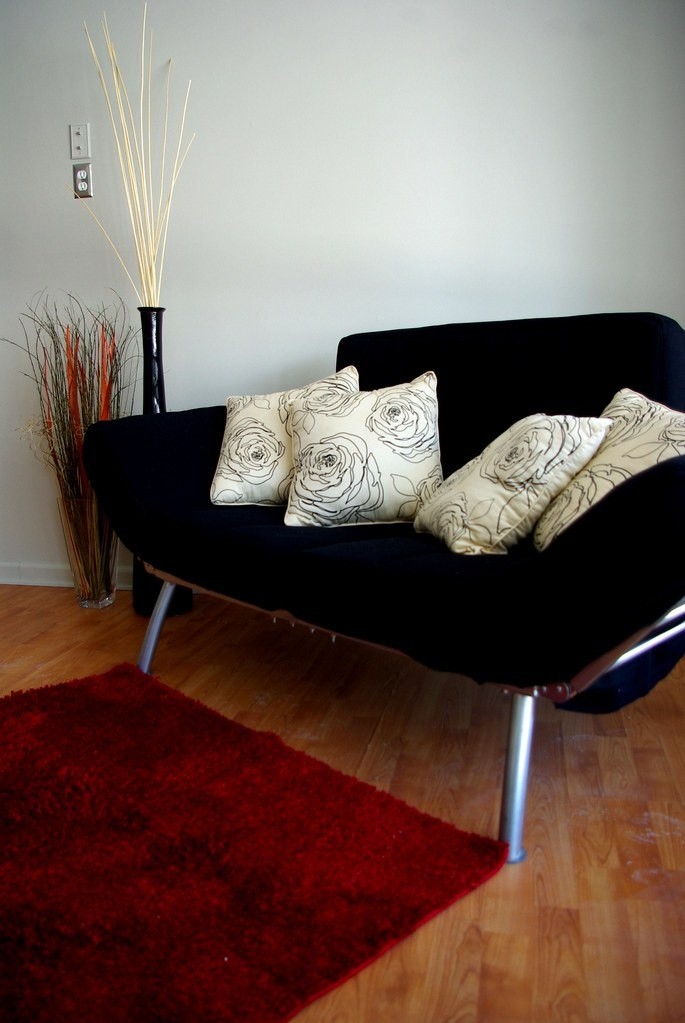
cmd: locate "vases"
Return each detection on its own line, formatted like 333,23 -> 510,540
57,498 -> 119,609
133,307 -> 193,616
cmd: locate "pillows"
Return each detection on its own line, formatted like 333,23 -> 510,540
533,388 -> 685,552
284,371 -> 443,528
209,365 -> 359,507
414,414 -> 613,556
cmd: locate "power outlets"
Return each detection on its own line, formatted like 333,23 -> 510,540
72,163 -> 94,200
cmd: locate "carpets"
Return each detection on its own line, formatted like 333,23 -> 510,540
0,664 -> 509,1022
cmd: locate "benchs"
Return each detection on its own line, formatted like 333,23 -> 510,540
82,312 -> 685,863
